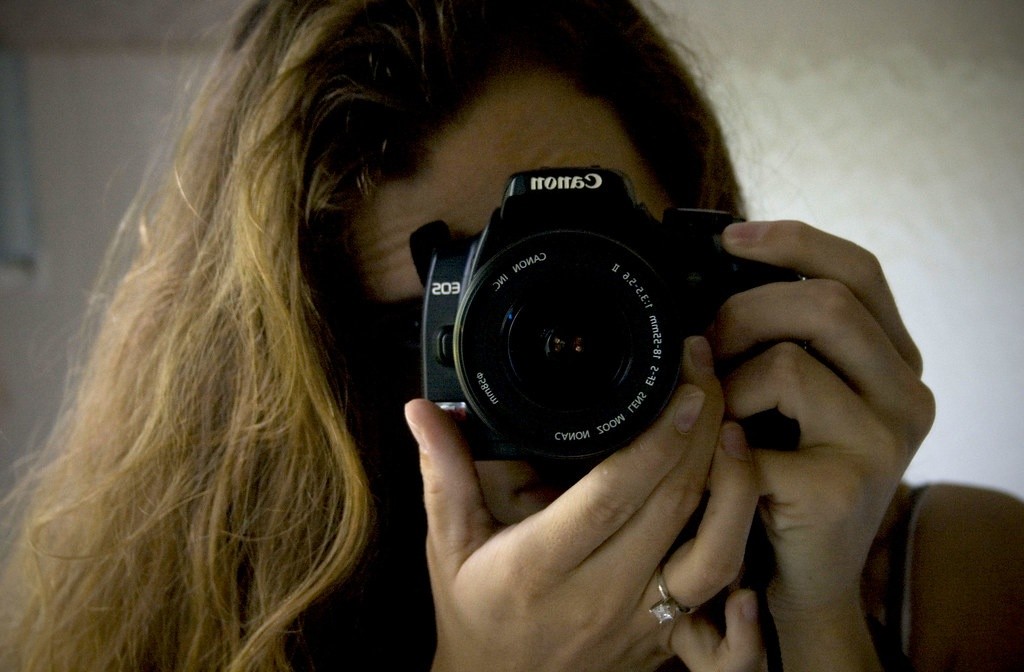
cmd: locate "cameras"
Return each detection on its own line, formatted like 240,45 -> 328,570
418,167 -> 801,466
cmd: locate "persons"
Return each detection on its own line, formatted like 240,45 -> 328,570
0,1 -> 1023,672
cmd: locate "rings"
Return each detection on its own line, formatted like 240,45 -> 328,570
648,565 -> 700,624
